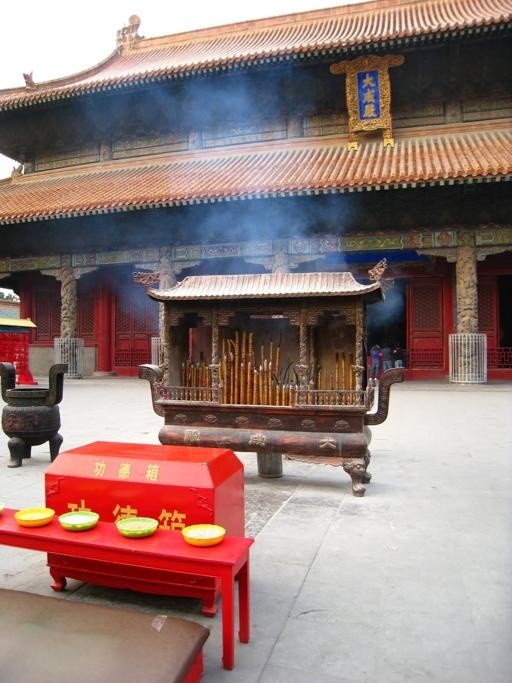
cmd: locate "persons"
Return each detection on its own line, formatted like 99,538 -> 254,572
392,344 -> 403,368
369,344 -> 382,379
382,341 -> 394,372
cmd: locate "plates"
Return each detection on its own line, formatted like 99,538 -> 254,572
13,505 -> 228,548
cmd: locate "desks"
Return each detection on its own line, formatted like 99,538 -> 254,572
1,509 -> 251,668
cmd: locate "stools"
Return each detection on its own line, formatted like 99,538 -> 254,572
1,586 -> 209,678
